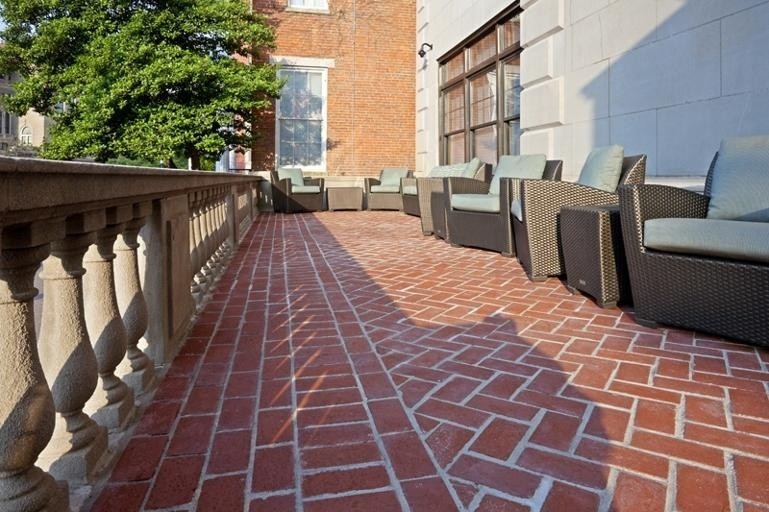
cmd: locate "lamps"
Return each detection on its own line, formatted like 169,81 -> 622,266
417,43 -> 433,58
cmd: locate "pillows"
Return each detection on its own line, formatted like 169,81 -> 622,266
487,154 -> 547,196
705,136 -> 769,222
459,157 -> 482,179
576,144 -> 625,193
380,167 -> 409,187
277,168 -> 304,186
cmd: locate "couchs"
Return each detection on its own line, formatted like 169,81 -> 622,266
270,170 -> 325,215
399,162 -> 487,219
618,150 -> 769,346
509,153 -> 648,282
442,160 -> 564,257
364,170 -> 413,211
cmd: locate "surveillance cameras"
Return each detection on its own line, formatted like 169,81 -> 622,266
419,50 -> 426,57
